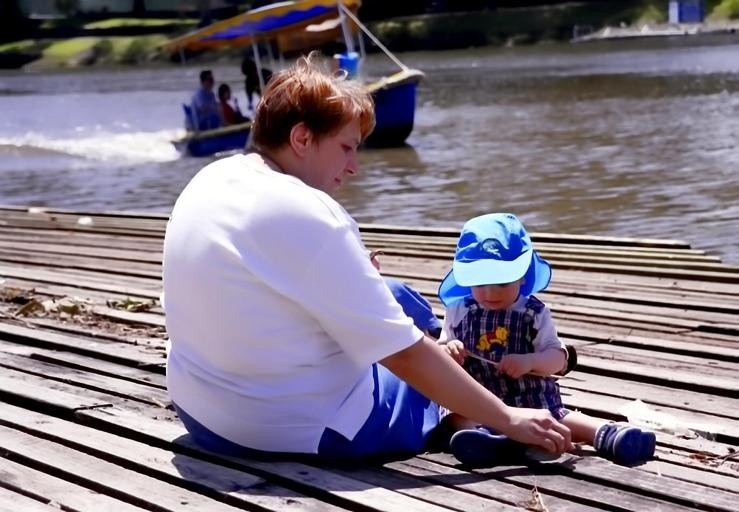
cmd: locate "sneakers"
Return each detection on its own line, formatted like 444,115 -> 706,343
592,419 -> 657,468
449,423 -> 528,469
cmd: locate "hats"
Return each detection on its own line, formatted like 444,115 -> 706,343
452,213 -> 534,288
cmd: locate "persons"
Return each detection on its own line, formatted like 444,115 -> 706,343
435,211 -> 656,468
241,57 -> 273,110
184,70 -> 217,131
218,83 -> 240,125
160,52 -> 579,474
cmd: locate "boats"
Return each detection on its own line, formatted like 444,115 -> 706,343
156,1 -> 425,157
